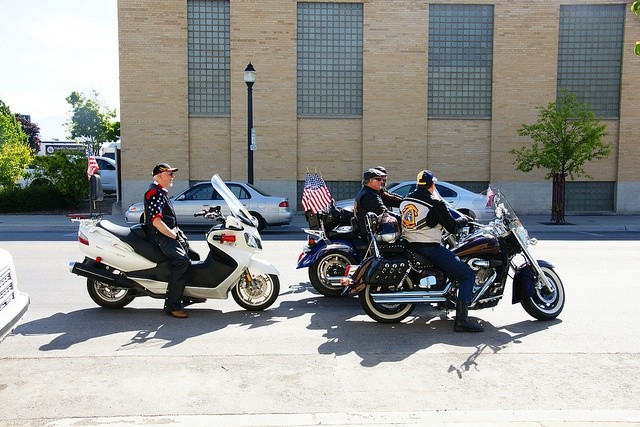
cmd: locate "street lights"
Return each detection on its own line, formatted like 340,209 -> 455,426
242,62 -> 256,185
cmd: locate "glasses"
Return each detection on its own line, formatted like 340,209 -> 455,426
375,178 -> 382,181
168,173 -> 173,176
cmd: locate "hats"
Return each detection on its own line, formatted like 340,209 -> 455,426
416,169 -> 437,185
375,166 -> 387,173
152,163 -> 178,177
364,168 -> 387,179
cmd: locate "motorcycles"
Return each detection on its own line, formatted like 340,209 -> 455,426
296,203 -> 473,295
68,173 -> 280,311
341,187 -> 566,320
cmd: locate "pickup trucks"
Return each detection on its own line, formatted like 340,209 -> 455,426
15,156 -> 117,192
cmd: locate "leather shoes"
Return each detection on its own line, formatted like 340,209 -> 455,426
173,310 -> 188,318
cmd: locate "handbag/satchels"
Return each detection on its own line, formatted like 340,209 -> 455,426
304,198 -> 341,231
363,258 -> 411,288
374,208 -> 400,243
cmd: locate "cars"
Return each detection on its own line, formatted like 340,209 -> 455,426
335,179 -> 495,222
124,180 -> 293,234
0,246 -> 32,344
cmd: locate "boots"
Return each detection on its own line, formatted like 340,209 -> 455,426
453,299 -> 485,332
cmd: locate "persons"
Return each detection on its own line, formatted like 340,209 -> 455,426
398,169 -> 485,333
352,168 -> 400,246
376,165 -> 404,211
142,162 -> 208,319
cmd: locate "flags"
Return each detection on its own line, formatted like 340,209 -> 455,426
308,173 -> 332,215
302,174 -> 313,213
86,144 -> 100,180
320,176 -> 330,214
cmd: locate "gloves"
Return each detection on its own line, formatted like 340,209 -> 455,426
176,233 -> 187,251
178,230 -> 189,248
